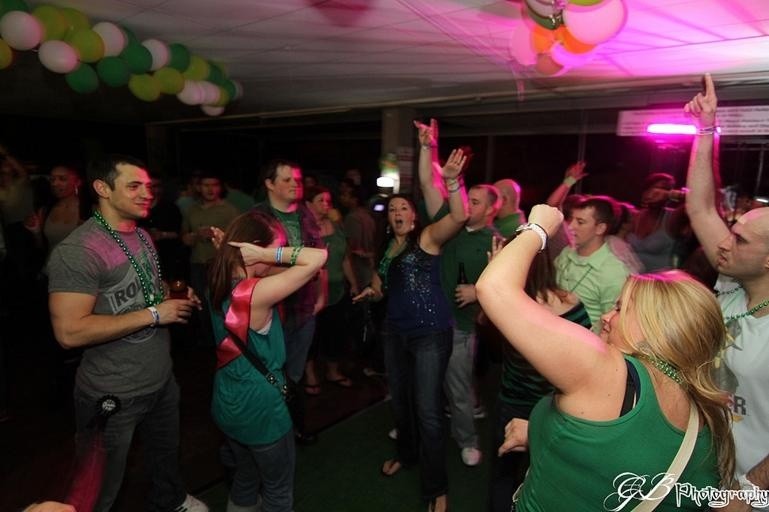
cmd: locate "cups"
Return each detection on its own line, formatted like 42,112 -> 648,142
168,281 -> 189,299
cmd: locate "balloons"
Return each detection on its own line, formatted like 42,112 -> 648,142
0,0 -> 244,117
506,1 -> 624,77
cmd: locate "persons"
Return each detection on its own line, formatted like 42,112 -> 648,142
683,71 -> 768,512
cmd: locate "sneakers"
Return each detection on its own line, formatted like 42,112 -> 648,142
443,403 -> 488,420
175,495 -> 209,512
461,447 -> 480,466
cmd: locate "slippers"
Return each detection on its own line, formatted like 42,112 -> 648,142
305,384 -> 320,397
333,377 -> 354,388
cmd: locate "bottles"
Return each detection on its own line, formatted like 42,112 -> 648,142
456,264 -> 467,304
